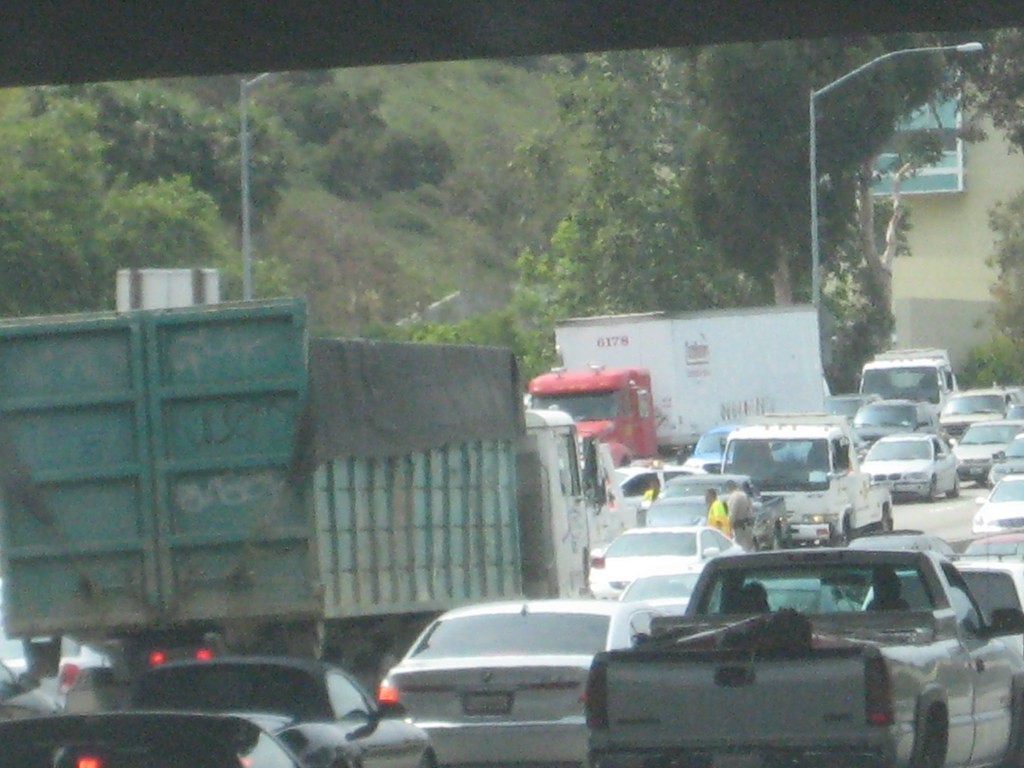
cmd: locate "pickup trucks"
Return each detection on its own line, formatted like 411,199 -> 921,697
580,542 -> 1024,768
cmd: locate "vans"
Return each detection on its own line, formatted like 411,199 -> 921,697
823,394 -> 884,426
851,398 -> 944,463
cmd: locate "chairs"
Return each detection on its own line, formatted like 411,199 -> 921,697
807,443 -> 837,472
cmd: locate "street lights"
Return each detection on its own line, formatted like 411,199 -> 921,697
810,41 -> 987,306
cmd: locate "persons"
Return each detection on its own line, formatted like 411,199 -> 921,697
726,479 -> 757,555
704,489 -> 731,540
639,484 -> 655,509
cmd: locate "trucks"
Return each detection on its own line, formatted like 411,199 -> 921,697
642,474 -> 790,551
720,411 -> 895,551
1,292 -> 606,659
856,347 -> 962,417
524,300 -> 830,478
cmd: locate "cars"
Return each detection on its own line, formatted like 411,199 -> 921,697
854,558 -> 1024,642
117,654 -> 440,768
970,471 -> 1024,535
859,444 -> 963,502
604,568 -> 830,656
374,598 -> 664,768
937,385 -> 1024,484
819,535 -> 960,611
613,466 -> 709,526
689,425 -> 743,472
0,631 -> 114,723
585,525 -> 749,597
1,709 -> 309,767
953,534 -> 1024,564
